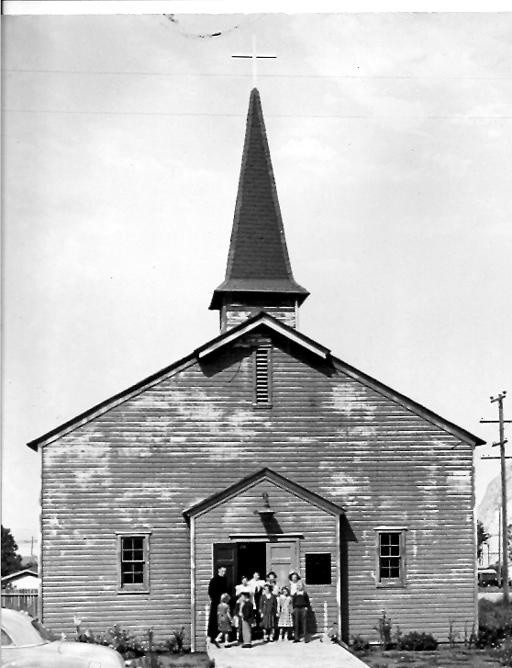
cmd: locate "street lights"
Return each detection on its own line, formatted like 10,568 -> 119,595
484,542 -> 489,566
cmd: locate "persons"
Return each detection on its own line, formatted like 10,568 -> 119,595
206,564 -> 311,648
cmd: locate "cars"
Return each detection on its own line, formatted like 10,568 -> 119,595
0,607 -> 126,668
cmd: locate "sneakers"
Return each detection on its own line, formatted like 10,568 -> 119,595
209,635 -> 310,648
